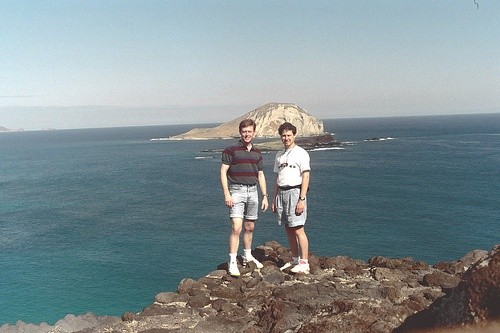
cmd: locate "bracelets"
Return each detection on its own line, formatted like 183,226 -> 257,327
262,194 -> 268,196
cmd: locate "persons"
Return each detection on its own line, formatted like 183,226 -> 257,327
272,122 -> 312,274
220,119 -> 269,276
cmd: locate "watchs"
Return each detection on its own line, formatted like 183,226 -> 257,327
299,196 -> 305,200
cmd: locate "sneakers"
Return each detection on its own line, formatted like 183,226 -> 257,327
279,256 -> 300,271
291,259 -> 310,274
242,256 -> 263,268
227,261 -> 241,277
